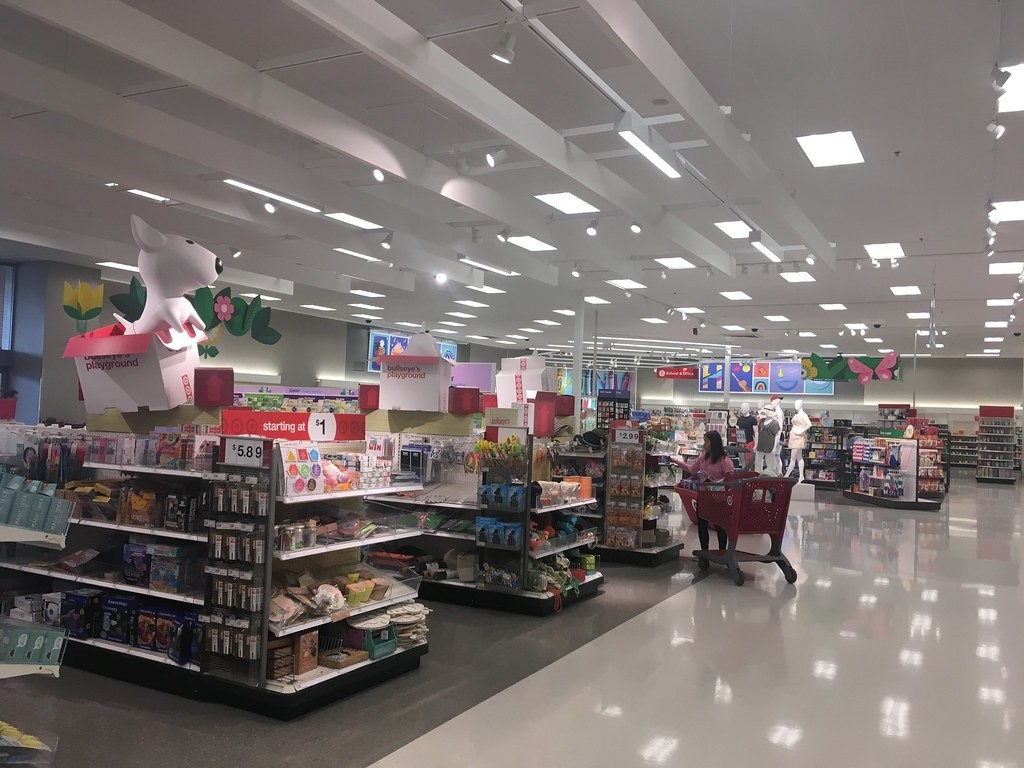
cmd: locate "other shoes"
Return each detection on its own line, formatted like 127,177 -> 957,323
690,556 -> 699,561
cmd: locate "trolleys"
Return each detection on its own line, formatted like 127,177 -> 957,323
674,470 -> 798,585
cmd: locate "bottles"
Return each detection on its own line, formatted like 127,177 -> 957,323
281,523 -> 316,551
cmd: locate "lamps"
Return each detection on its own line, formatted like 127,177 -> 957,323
658,271 -> 668,281
706,267 -> 715,278
586,213 -> 602,237
623,291 -> 631,299
485,147 -> 508,167
982,66 -> 1024,325
570,261 -> 585,278
263,202 -> 279,214
380,231 -> 394,250
794,264 -> 802,274
497,226 -> 511,243
761,263 -> 771,276
805,253 -> 901,272
776,263 -> 782,273
741,266 -> 750,276
630,221 -> 644,234
470,226 -> 485,244
228,246 -> 242,258
664,307 -> 708,330
785,329 -> 949,336
540,342 -> 757,372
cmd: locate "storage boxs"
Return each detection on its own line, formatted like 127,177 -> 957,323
495,355 -> 559,409
121,541 -> 205,597
14,592 -> 46,612
130,603 -> 204,668
314,554 -> 391,609
449,387 -> 480,415
534,392 -> 575,436
512,402 -> 535,434
99,595 -> 133,646
62,587 -> 104,640
62,323 -> 208,415
478,392 -> 497,416
358,383 -> 379,410
194,367 -> 235,408
164,493 -> 197,533
367,355 -> 455,413
268,647 -> 293,677
41,594 -> 62,628
10,608 -> 43,622
342,545 -> 423,599
293,630 -> 319,675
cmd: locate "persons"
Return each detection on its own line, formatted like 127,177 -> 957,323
758,394 -> 785,476
783,399 -> 812,483
668,430 -> 736,564
730,403 -> 758,469
754,404 -> 781,476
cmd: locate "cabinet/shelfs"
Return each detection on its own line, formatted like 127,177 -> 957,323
899,424 -> 950,499
1014,427 -> 1022,467
950,434 -> 977,465
802,419 -> 877,492
549,420 -> 684,566
974,405 -> 1017,485
362,415 -> 605,618
0,412 -> 429,725
840,418 -> 942,511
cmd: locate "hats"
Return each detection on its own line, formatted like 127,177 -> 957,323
759,405 -> 777,418
770,395 -> 784,402
553,424 -> 607,451
